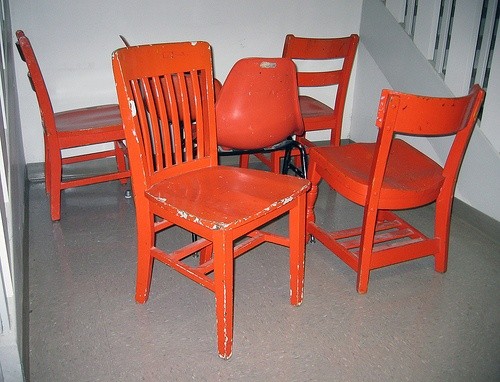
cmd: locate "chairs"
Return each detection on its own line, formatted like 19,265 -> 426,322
177,57 -> 316,258
16,30 -> 134,220
238,32 -> 360,179
305,84 -> 486,295
120,35 -> 222,172
111,41 -> 311,360
